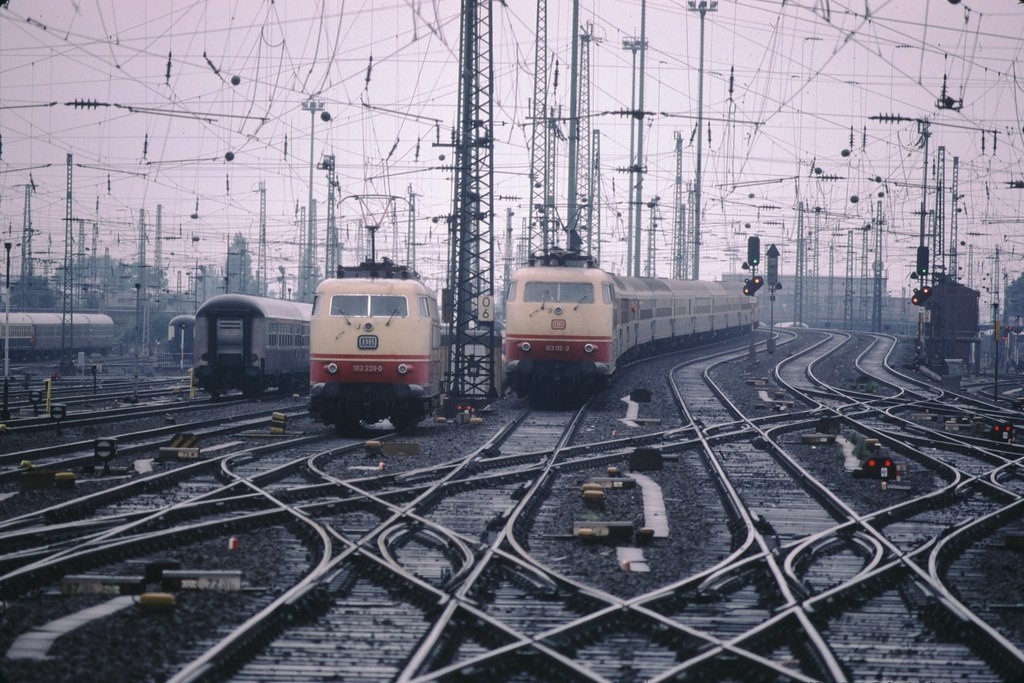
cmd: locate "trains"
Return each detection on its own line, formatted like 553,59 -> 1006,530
303,258 -> 445,438
503,266 -> 759,398
2,293 -> 313,400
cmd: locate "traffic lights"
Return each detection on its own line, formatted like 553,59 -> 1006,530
911,286 -> 933,306
917,247 -> 929,276
748,237 -> 760,264
743,276 -> 764,295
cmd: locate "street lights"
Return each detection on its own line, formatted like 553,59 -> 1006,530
2,241 -> 12,412
